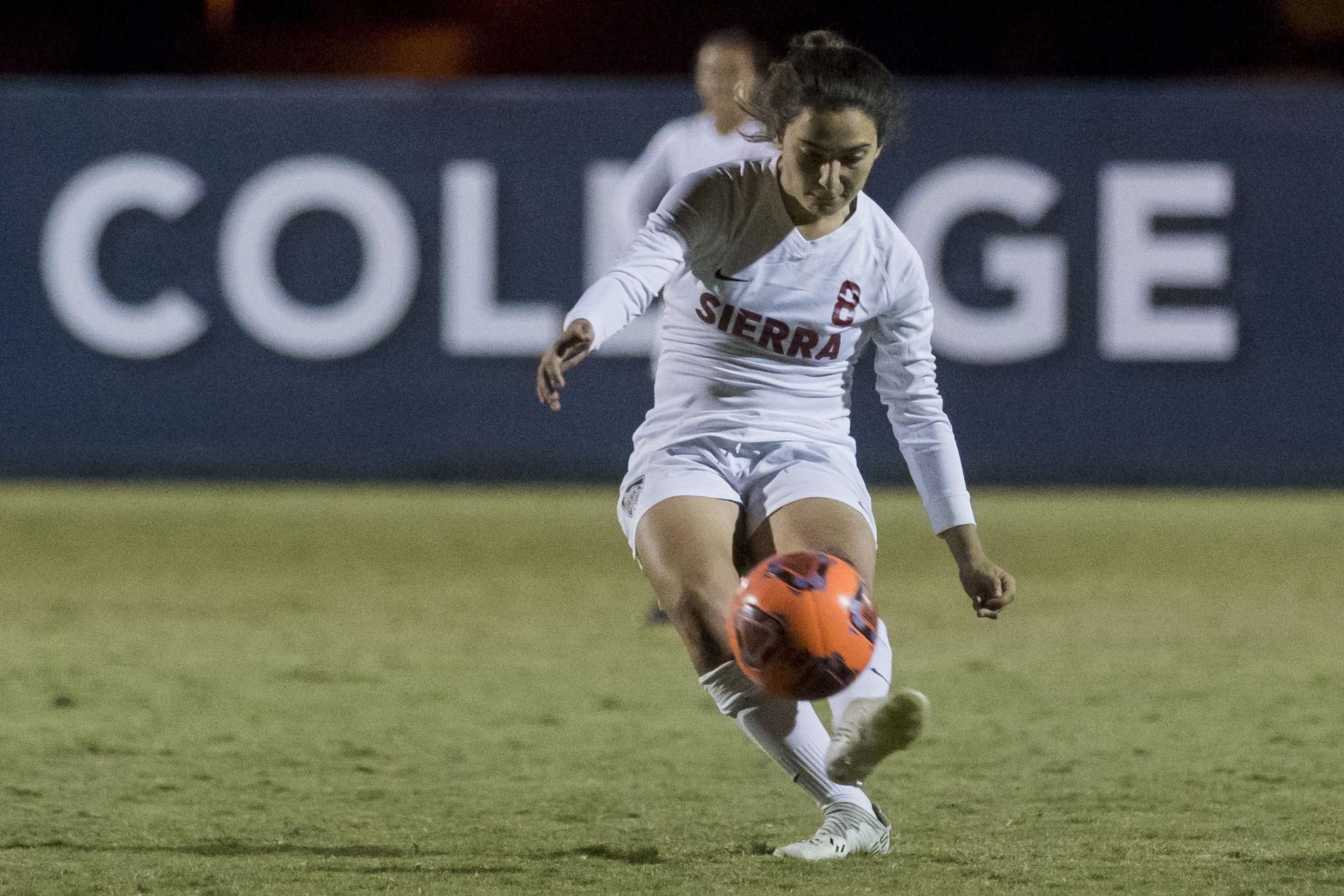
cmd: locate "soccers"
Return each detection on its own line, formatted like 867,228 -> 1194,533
728,549 -> 876,699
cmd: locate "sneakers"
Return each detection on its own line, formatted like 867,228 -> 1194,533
824,688 -> 931,788
772,802 -> 892,864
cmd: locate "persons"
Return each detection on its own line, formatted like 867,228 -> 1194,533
616,32 -> 779,246
534,28 -> 1018,863
